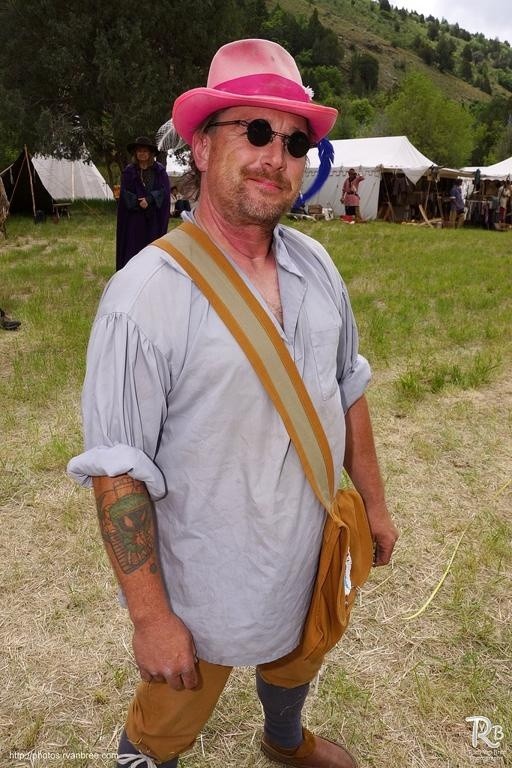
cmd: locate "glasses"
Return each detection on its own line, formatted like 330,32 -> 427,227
205,117 -> 316,159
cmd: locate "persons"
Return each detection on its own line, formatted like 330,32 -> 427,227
66,36 -> 401,767
339,168 -> 366,221
0,165 -> 21,336
115,135 -> 170,272
169,186 -> 191,219
450,177 -> 466,221
496,178 -> 512,223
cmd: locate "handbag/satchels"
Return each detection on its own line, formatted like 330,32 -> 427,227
299,488 -> 375,664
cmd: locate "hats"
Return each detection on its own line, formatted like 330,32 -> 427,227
171,36 -> 340,147
126,136 -> 160,157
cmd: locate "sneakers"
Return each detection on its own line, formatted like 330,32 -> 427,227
259,726 -> 358,768
0,315 -> 22,331
